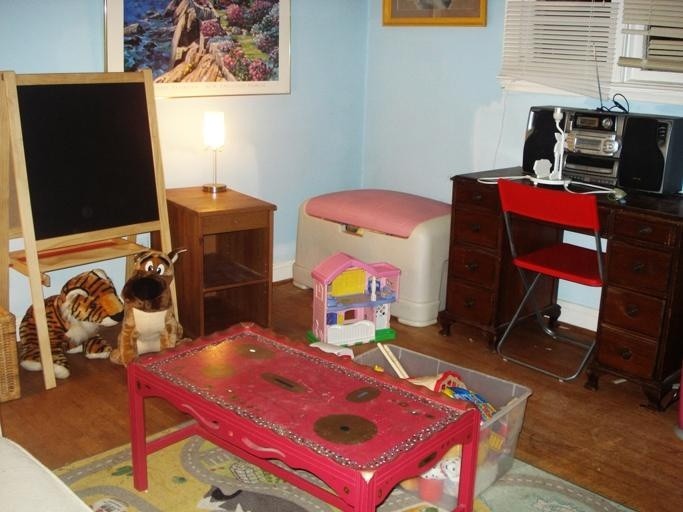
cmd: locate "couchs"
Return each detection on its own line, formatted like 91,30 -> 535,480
0,428 -> 93,512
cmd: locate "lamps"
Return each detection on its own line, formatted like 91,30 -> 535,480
203,111 -> 227,193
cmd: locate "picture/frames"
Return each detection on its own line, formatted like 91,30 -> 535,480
383,0 -> 486,26
105,0 -> 291,97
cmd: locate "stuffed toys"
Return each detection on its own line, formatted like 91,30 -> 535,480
18,269 -> 124,379
110,248 -> 193,367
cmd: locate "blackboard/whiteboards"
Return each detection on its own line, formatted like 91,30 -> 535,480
1,68 -> 168,251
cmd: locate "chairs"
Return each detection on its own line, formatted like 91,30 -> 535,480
494,179 -> 605,380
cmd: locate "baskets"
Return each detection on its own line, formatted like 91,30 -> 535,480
0,307 -> 22,402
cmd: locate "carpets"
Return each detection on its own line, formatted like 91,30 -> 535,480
50,416 -> 635,512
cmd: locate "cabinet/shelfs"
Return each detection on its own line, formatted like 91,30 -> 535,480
166,187 -> 277,340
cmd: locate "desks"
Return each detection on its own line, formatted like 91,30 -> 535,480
439,167 -> 683,410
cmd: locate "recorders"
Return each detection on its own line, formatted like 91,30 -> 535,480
522,106 -> 682,194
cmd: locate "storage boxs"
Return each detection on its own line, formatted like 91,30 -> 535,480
352,345 -> 532,512
294,190 -> 449,328
